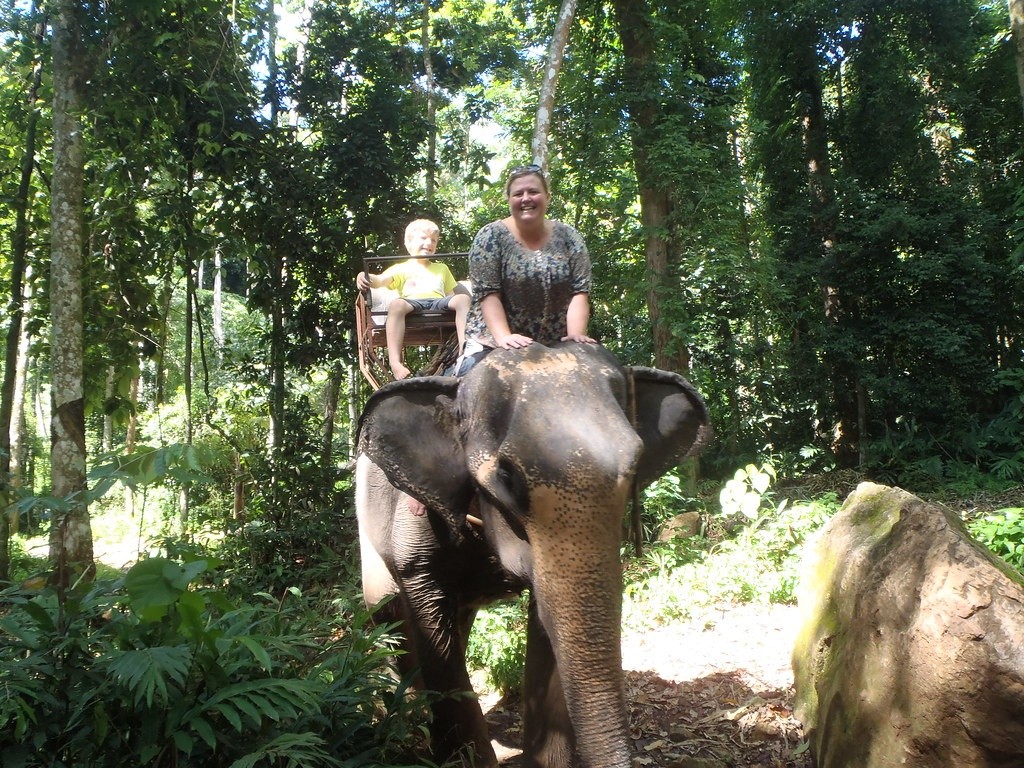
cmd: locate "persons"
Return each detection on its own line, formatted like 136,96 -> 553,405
357,218 -> 473,380
458,167 -> 597,376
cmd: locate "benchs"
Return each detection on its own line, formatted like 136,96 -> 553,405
355,290 -> 456,391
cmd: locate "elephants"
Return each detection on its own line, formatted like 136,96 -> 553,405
354,336 -> 706,768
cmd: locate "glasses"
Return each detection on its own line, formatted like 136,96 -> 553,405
509,164 -> 544,175
